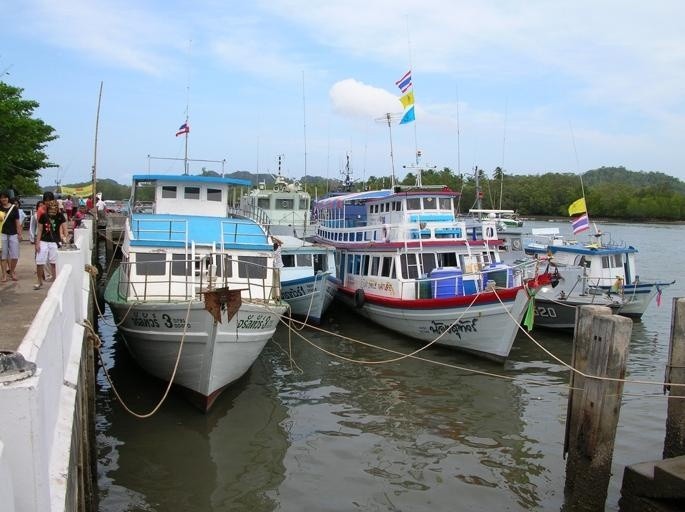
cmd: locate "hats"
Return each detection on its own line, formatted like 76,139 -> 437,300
0,350 -> 36,382
43,192 -> 54,200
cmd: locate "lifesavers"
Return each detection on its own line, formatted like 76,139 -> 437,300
380,224 -> 390,243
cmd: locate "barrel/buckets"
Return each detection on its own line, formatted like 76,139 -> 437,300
430,266 -> 462,298
483,261 -> 513,291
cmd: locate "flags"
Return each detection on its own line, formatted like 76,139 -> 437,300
399,92 -> 414,110
571,216 -> 590,235
176,123 -> 189,138
568,198 -> 586,217
395,70 -> 412,93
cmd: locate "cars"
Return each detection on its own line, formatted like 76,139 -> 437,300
16,193 -> 49,230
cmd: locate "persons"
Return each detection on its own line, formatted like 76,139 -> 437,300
0,191 -> 27,282
29,192 -> 107,289
